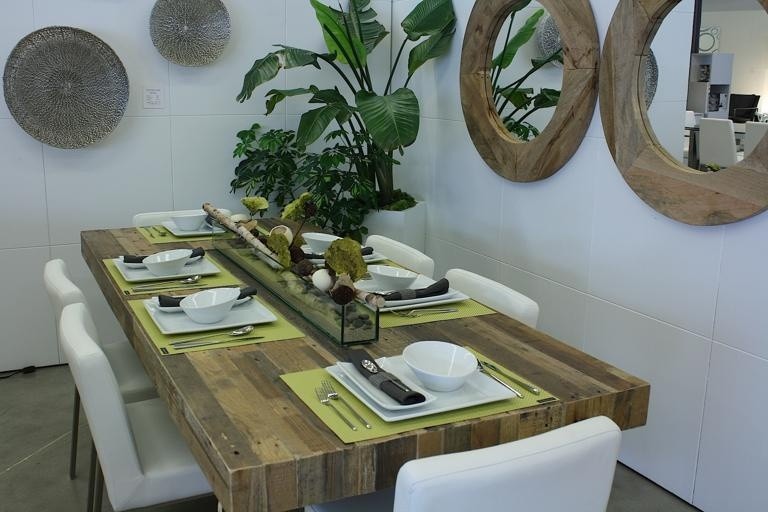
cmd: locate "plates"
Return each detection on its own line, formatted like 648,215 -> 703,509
161,219 -> 226,239
142,291 -> 278,336
325,356 -> 517,424
116,258 -> 222,284
346,270 -> 472,315
300,246 -> 388,268
339,357 -> 436,412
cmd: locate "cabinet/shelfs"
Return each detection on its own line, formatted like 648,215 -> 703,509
686,52 -> 732,119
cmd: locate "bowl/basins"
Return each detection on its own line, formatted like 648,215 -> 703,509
301,232 -> 343,255
172,214 -> 207,231
401,340 -> 479,395
180,286 -> 239,324
368,263 -> 416,292
142,248 -> 191,276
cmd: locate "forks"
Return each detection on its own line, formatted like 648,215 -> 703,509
323,378 -> 374,432
388,307 -> 459,319
310,387 -> 356,433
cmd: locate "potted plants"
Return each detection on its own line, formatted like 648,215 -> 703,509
233,2 -> 457,252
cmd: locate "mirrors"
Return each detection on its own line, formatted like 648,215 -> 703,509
456,0 -> 599,186
600,0 -> 767,230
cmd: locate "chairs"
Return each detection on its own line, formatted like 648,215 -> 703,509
681,90 -> 767,174
391,415 -> 624,512
130,207 -> 230,232
58,303 -> 218,512
41,255 -> 162,512
363,233 -> 439,278
446,269 -> 540,331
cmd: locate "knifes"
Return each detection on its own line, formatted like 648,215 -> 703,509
478,359 -> 540,396
175,338 -> 265,353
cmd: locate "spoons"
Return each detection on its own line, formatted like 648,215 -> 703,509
170,326 -> 256,346
145,227 -> 166,239
133,275 -> 203,292
